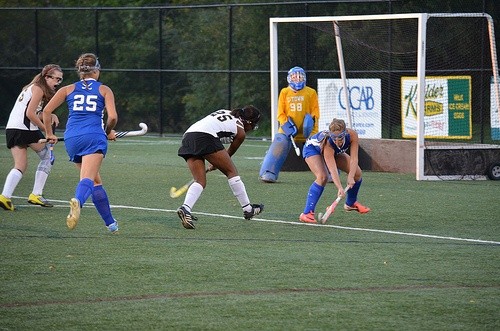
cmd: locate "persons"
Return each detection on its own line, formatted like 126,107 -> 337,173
299,118 -> 370,222
176,105 -> 264,230
0,64 -> 64,210
43,53 -> 118,235
259,67 -> 340,183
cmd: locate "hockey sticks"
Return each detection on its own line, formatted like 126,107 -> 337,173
289,135 -> 300,156
169,169 -> 209,198
317,186 -> 350,224
38,122 -> 149,144
50,122 -> 55,165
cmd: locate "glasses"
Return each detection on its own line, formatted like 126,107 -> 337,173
330,130 -> 347,140
48,75 -> 63,82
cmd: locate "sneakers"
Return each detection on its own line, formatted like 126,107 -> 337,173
300,211 -> 318,223
27,193 -> 52,207
177,206 -> 196,229
243,203 -> 264,220
344,201 -> 371,214
0,194 -> 16,211
107,218 -> 118,233
66,198 -> 81,229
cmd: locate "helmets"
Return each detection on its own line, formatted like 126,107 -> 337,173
287,66 -> 306,90
